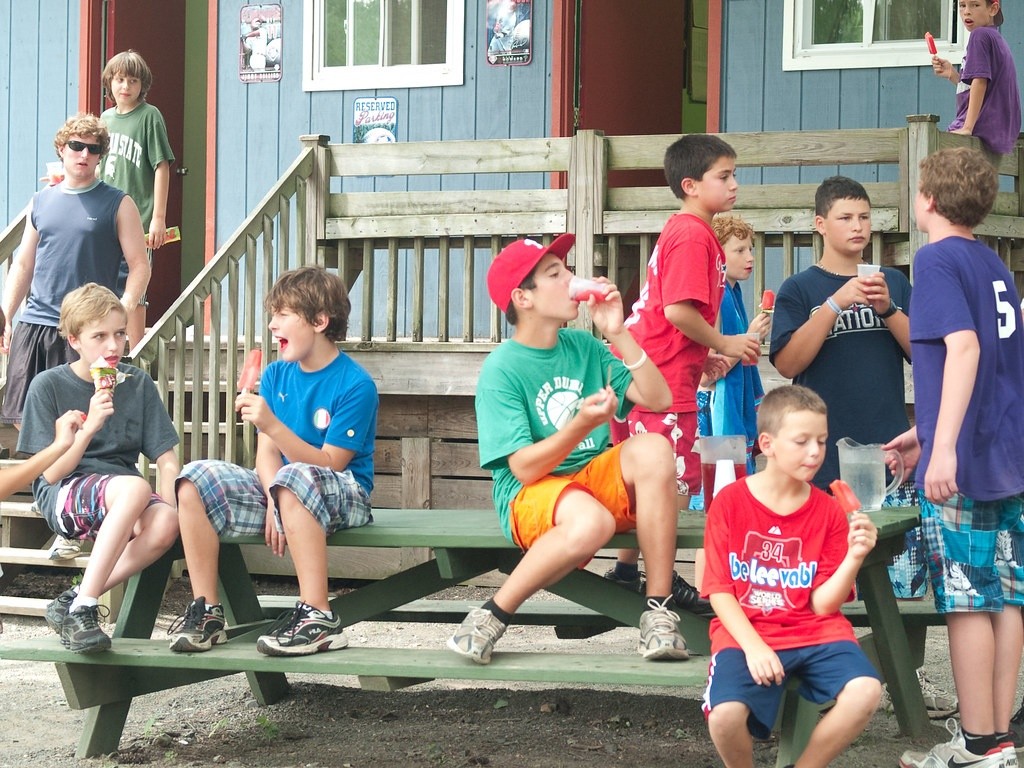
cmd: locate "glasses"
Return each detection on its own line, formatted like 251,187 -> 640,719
65,140 -> 103,154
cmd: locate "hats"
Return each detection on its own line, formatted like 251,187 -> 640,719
991,0 -> 1003,26
486,234 -> 576,315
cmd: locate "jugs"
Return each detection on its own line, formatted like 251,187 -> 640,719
835,436 -> 904,514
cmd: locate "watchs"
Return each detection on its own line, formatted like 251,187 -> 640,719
873,299 -> 898,319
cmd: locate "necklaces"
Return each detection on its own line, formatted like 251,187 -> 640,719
816,262 -> 869,276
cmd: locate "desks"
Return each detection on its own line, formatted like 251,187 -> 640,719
56,507 -> 933,768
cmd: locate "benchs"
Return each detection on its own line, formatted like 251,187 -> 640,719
255,594 -> 946,628
0,636 -> 708,686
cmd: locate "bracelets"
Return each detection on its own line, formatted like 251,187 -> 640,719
828,297 -> 843,315
624,350 -> 647,369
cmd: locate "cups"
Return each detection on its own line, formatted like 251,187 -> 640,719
737,333 -> 760,366
857,263 -> 881,279
568,275 -> 610,304
46,162 -> 62,186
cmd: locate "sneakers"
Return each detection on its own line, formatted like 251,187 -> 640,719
257,600 -> 349,656
639,570 -> 716,615
637,593 -> 690,660
60,604 -> 111,654
47,535 -> 85,560
447,606 -> 506,664
31,500 -> 42,515
898,718 -> 1019,768
1009,698 -> 1024,752
167,596 -> 227,652
605,569 -> 642,594
885,667 -> 959,720
44,583 -> 80,636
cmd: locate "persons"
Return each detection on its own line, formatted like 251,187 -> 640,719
0,410 -> 85,502
769,175 -> 960,722
37,49 -> 178,362
882,149 -> 1024,768
700,385 -> 877,768
448,233 -> 689,665
0,114 -> 151,561
602,134 -> 762,619
16,282 -> 184,655
932,0 -> 1022,154
688,217 -> 771,593
168,266 -> 380,656
